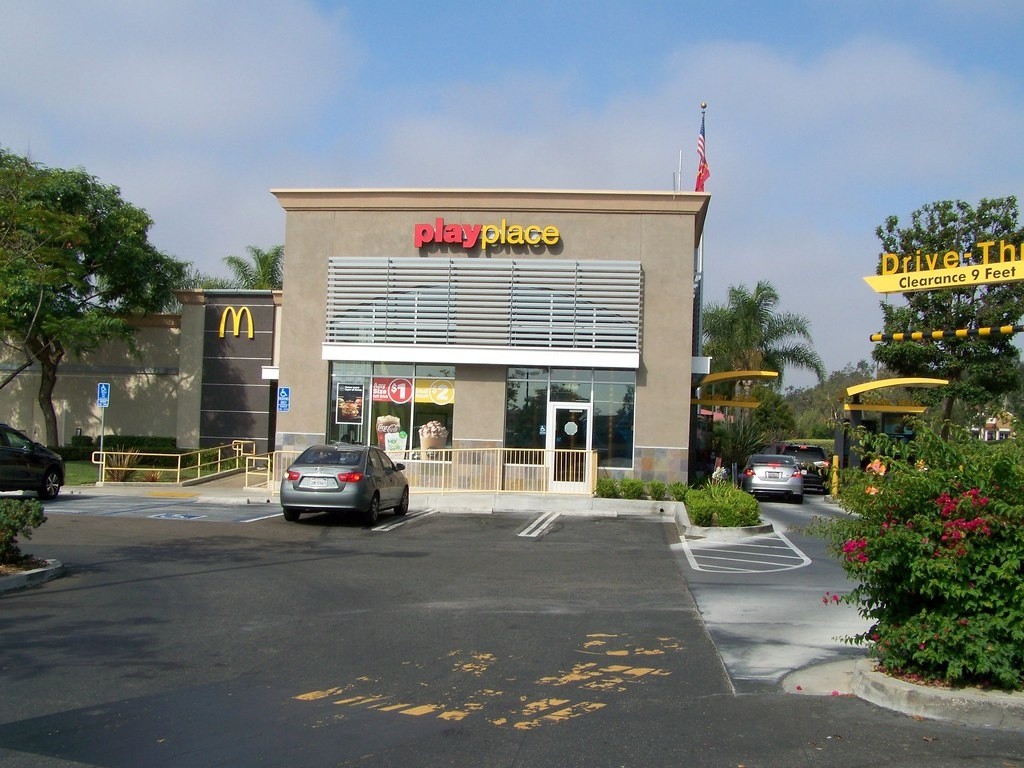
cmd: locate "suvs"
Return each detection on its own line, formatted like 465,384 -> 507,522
775,443 -> 832,496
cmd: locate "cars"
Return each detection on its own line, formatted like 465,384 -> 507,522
279,442 -> 410,526
740,453 -> 804,504
0,423 -> 65,500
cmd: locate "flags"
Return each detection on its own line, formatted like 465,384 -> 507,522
695,111 -> 710,193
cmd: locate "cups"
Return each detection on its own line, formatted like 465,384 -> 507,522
417,432 -> 449,461
376,417 -> 401,451
385,433 -> 408,459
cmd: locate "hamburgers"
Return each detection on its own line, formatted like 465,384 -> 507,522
341,403 -> 359,417
